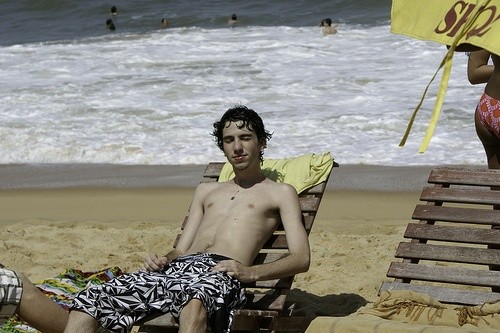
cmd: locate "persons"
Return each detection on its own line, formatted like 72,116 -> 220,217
227,13 -> 239,27
0,106 -> 312,333
161,18 -> 170,28
105,5 -> 118,31
319,18 -> 337,37
467,50 -> 500,293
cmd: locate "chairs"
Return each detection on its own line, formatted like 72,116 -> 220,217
304,167 -> 500,333
129,160 -> 339,333
